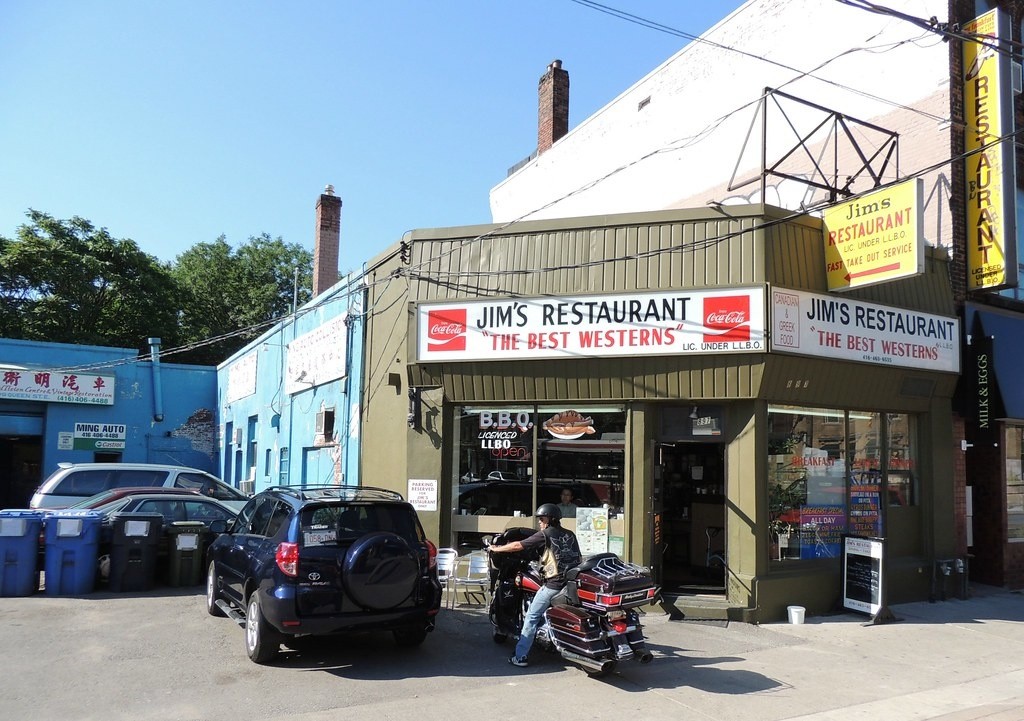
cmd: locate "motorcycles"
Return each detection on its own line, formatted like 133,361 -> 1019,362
462,527 -> 663,679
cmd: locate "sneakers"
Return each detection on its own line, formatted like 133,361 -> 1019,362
507,656 -> 528,666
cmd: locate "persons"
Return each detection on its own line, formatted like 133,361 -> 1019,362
556,489 -> 577,517
487,504 -> 582,666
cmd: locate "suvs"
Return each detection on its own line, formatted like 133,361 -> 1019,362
205,484 -> 443,664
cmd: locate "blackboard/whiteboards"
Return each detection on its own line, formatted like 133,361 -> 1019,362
840,533 -> 888,616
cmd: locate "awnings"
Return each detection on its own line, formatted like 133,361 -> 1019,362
951,308 -> 1024,419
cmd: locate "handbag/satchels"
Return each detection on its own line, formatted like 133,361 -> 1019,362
542,548 -> 559,579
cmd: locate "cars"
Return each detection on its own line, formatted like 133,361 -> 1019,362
460,472 -> 481,484
459,482 -> 602,517
40,493 -> 242,589
485,471 -> 522,482
778,485 -> 907,543
30,461 -> 251,517
782,477 -> 854,504
69,487 -> 201,510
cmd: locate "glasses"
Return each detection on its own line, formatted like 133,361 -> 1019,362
539,518 -> 550,522
560,493 -> 571,496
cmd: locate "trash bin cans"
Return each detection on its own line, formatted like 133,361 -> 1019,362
0,506 -> 46,598
39,509 -> 104,600
109,514 -> 166,596
162,521 -> 214,591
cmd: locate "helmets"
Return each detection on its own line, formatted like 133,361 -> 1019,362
533,503 -> 562,520
